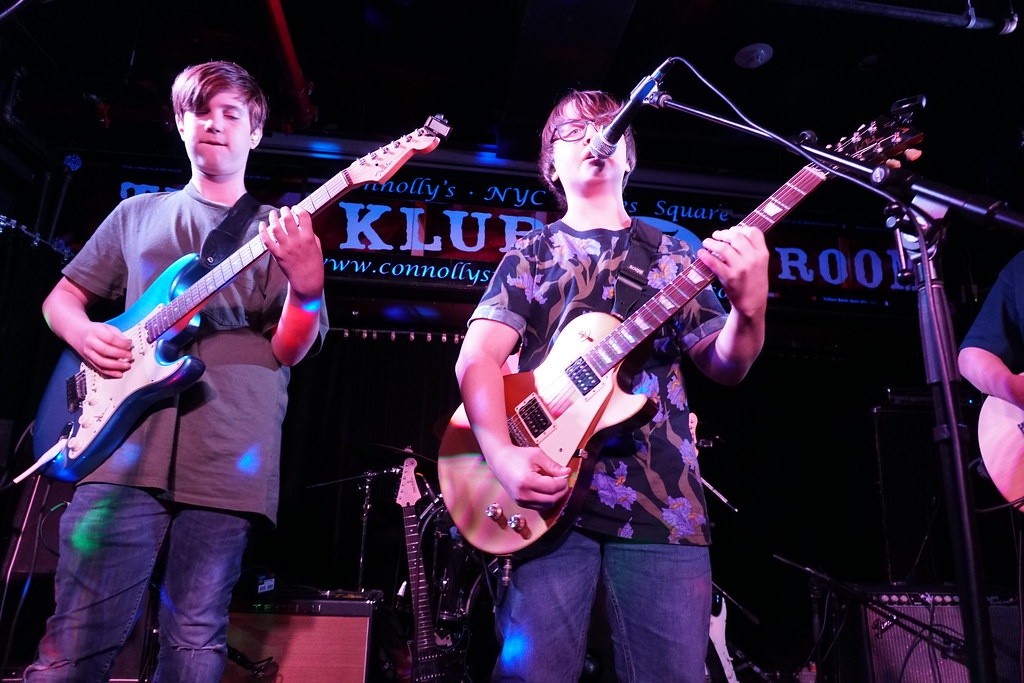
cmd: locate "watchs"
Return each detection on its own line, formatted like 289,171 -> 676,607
289,286 -> 324,313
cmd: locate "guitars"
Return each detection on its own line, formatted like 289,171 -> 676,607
976,369 -> 1024,518
437,88 -> 928,555
388,456 -> 469,683
31,116 -> 454,483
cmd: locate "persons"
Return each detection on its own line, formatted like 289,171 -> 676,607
22,58 -> 332,682
956,245 -> 1024,415
451,87 -> 771,683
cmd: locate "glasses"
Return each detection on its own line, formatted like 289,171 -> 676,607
549,118 -> 605,142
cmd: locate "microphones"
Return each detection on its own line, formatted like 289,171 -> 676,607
247,665 -> 265,683
589,57 -> 675,160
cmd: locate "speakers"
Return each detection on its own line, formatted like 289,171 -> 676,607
217,600 -> 372,683
106,587 -> 153,683
830,583 -> 1024,683
874,409 -> 1018,588
1,465 -> 77,578
